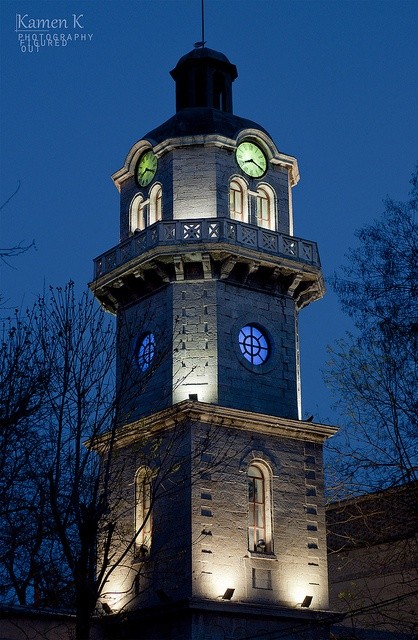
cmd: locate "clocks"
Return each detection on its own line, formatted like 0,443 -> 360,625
134,148 -> 158,188
235,141 -> 268,179
